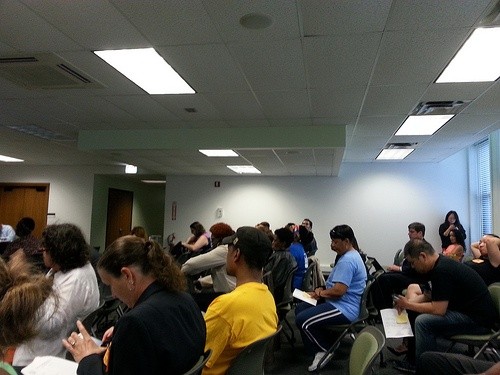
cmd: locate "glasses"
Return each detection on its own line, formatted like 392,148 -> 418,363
331,230 -> 346,238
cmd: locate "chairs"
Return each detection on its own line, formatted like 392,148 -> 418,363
32,257 -> 500,375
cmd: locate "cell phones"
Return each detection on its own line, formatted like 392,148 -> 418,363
394,295 -> 400,300
77,332 -> 103,347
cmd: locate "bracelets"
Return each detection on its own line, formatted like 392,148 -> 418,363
319,290 -> 322,297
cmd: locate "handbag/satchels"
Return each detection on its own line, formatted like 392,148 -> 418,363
171,241 -> 188,260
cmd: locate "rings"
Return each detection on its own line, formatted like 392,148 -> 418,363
71,341 -> 75,345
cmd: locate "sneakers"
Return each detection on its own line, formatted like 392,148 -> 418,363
392,362 -> 416,373
308,351 -> 333,371
331,342 -> 340,352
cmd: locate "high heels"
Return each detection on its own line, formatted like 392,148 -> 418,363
387,346 -> 408,356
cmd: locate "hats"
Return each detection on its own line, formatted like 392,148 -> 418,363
222,227 -> 274,259
209,222 -> 235,240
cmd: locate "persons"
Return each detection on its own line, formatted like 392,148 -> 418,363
294,225 -> 367,371
202,226 -> 278,375
1,224 -> 100,375
442,229 -> 466,263
438,210 -> 467,254
414,351 -> 500,375
62,235 -> 206,375
396,238 -> 492,370
393,234 -> 500,354
376,222 -> 427,323
2,217 -> 318,323
